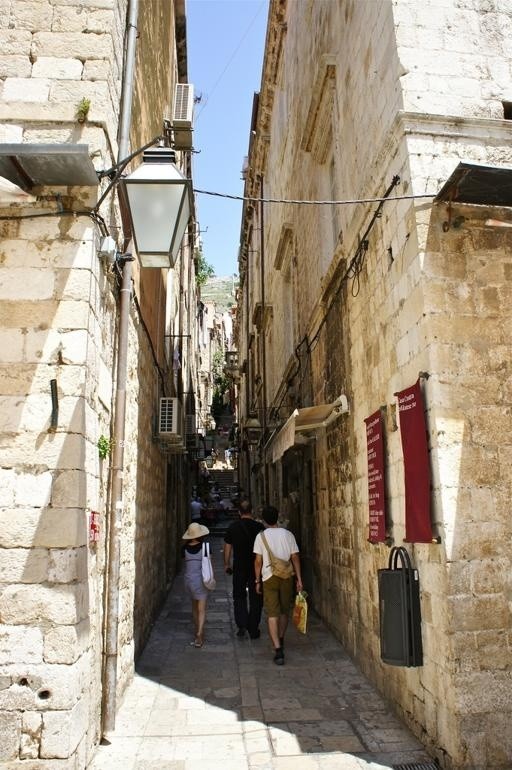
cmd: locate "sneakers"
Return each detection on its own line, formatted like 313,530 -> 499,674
273,647 -> 284,665
236,627 -> 260,639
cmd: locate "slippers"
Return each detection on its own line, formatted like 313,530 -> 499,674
190,639 -> 203,647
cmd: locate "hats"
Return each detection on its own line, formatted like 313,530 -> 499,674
182,522 -> 210,540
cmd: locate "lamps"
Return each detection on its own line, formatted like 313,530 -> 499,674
95,135 -> 197,269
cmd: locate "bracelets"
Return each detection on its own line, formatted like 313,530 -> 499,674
255,580 -> 260,584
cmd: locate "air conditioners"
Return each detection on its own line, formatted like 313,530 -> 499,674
172,84 -> 195,151
158,396 -> 187,453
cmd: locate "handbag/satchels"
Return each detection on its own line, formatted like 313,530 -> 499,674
270,556 -> 294,579
201,556 -> 216,591
292,595 -> 308,634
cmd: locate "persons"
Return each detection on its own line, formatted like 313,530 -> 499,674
223,499 -> 267,641
181,522 -> 217,649
188,481 -> 235,522
252,506 -> 304,666
